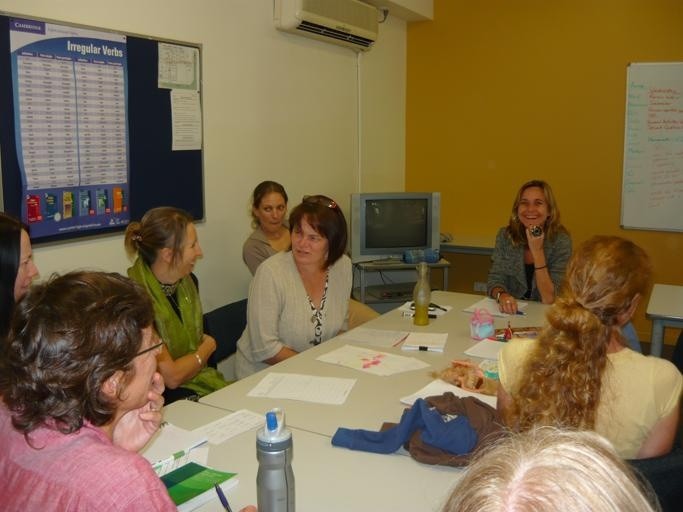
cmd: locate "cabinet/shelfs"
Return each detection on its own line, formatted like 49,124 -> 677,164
347,250 -> 451,315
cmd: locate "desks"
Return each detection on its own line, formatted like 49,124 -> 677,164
195,289 -> 558,512
644,282 -> 683,357
104,398 -> 471,512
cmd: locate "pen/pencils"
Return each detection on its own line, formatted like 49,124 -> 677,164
214,482 -> 231,511
430,302 -> 448,313
516,310 -> 526,315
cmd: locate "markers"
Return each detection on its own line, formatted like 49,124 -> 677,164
402,311 -> 439,319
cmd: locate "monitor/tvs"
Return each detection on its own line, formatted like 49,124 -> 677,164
351,191 -> 440,264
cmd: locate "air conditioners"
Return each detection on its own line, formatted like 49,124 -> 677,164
272,1 -> 379,53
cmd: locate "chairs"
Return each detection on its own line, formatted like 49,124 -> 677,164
202,299 -> 248,371
622,327 -> 683,511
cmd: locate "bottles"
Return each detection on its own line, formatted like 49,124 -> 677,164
256,408 -> 294,512
404,247 -> 445,263
413,261 -> 430,326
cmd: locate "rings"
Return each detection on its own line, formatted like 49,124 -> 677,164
529,222 -> 544,237
505,300 -> 509,304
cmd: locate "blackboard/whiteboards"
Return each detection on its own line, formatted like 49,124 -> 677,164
618,61 -> 682,233
1,10 -> 206,248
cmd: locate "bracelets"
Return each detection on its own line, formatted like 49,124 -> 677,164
188,350 -> 204,370
533,264 -> 548,270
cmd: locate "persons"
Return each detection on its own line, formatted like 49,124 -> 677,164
486,180 -> 573,315
232,195 -> 352,381
436,418 -> 663,512
241,180 -> 381,337
124,208 -> 237,407
0,272 -> 256,512
497,237 -> 683,460
0,211 -> 38,332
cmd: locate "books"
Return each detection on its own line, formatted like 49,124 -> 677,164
160,461 -> 239,512
402,332 -> 449,352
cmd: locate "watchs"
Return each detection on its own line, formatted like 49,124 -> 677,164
496,290 -> 507,302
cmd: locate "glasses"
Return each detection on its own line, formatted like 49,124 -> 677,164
303,194 -> 348,229
119,338 -> 165,369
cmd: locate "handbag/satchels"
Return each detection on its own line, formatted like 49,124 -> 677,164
469,306 -> 497,341
